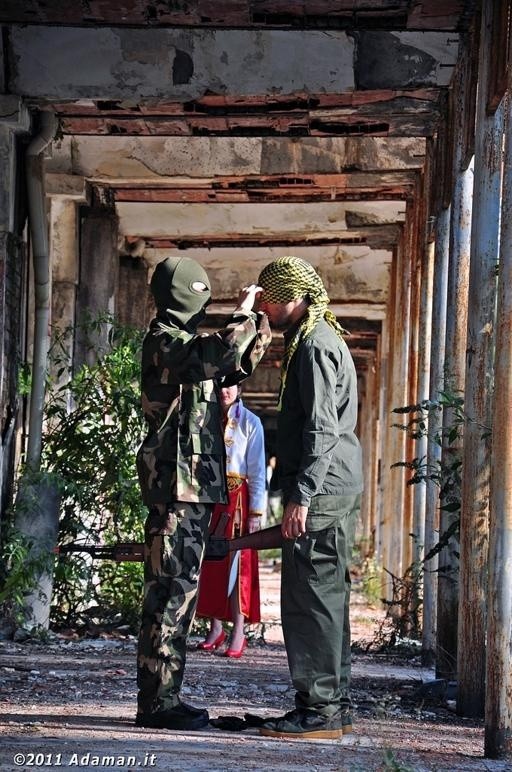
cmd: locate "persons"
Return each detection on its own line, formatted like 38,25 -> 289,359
132,254 -> 273,727
193,384 -> 268,656
258,255 -> 364,740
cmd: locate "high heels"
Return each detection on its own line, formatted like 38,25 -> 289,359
197,628 -> 225,650
224,638 -> 247,658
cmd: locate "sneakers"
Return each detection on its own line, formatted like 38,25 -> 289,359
260,709 -> 353,739
135,702 -> 209,730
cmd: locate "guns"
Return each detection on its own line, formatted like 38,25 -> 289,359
53,512 -> 283,561
